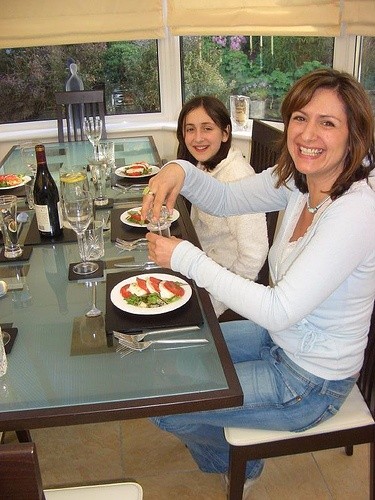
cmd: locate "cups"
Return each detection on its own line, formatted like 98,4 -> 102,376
20,142 -> 41,179
25,179 -> 35,209
77,221 -> 104,261
0,327 -> 7,376
230,95 -> 249,130
0,195 -> 22,258
88,160 -> 108,206
98,140 -> 115,168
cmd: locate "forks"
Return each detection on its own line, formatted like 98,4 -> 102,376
119,338 -> 208,351
115,242 -> 148,250
116,238 -> 147,245
113,326 -> 200,341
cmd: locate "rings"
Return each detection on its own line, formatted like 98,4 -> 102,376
142,187 -> 155,196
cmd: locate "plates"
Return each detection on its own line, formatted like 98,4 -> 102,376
115,166 -> 160,178
120,206 -> 180,227
0,176 -> 31,189
110,273 -> 192,315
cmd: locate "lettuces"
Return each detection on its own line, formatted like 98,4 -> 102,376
127,210 -> 146,225
123,292 -> 180,305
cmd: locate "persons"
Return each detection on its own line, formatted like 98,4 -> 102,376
140,67 -> 375,500
176,96 -> 270,322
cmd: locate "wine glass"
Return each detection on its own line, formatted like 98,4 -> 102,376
63,191 -> 99,275
85,120 -> 102,160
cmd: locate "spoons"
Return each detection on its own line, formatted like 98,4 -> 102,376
17,212 -> 28,235
87,284 -> 102,316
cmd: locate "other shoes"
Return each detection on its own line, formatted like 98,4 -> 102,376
226,459 -> 265,500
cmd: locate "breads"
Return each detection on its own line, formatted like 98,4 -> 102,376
0,174 -> 22,187
124,161 -> 149,173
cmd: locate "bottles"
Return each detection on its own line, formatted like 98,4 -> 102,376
33,145 -> 63,239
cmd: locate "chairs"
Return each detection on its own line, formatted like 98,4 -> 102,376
224,293 -> 375,500
55,91 -> 107,142
212,117 -> 295,323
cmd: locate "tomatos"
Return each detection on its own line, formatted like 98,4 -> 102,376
120,277 -> 184,297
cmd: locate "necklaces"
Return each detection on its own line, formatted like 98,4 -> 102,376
305,189 -> 331,213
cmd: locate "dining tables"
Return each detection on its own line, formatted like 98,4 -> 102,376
0,134 -> 244,433
